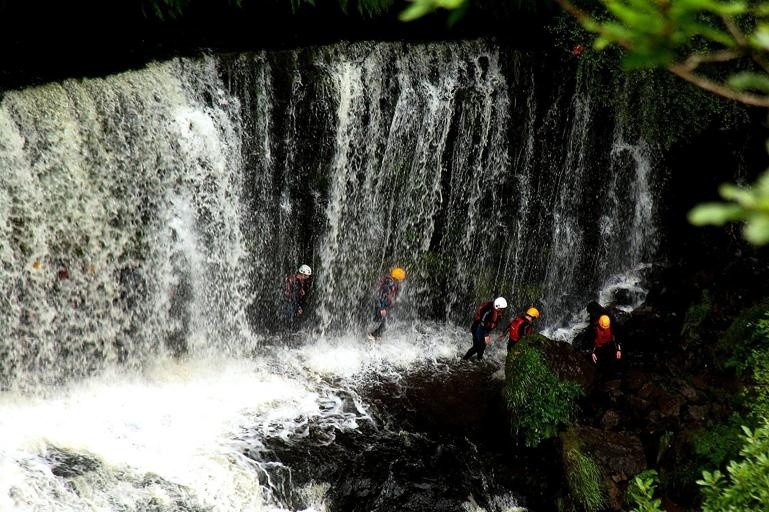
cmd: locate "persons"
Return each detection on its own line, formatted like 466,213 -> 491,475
497,307 -> 541,363
365,266 -> 407,342
589,314 -> 623,371
284,263 -> 312,326
461,295 -> 509,363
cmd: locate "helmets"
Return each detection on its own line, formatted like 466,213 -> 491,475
390,267 -> 406,280
526,307 -> 540,319
598,314 -> 611,329
298,264 -> 312,276
493,296 -> 508,310
